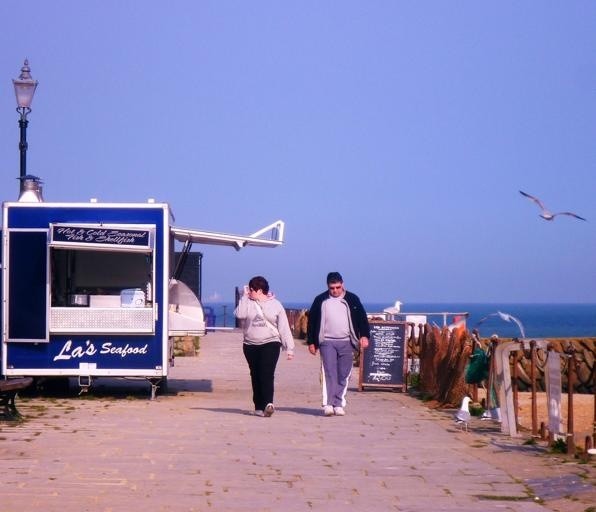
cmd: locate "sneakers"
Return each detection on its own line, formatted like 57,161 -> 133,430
255,410 -> 264,417
323,405 -> 334,416
264,403 -> 274,416
334,406 -> 345,416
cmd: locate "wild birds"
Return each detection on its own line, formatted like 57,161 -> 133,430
455,396 -> 474,434
383,300 -> 404,321
519,190 -> 589,222
476,310 -> 526,339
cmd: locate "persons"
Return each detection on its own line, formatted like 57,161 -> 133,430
232,276 -> 296,417
306,271 -> 371,415
